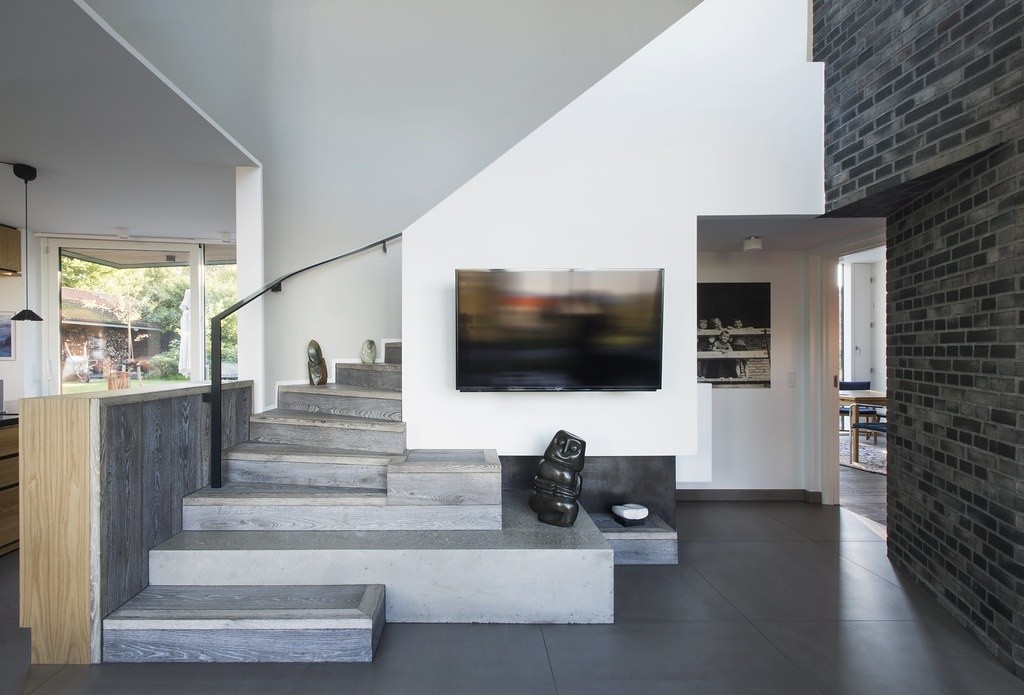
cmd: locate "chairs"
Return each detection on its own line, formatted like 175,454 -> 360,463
839,381 -> 888,445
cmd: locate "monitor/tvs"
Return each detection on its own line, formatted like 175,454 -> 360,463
456,269 -> 665,393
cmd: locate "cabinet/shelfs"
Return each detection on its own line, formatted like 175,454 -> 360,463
0,424 -> 20,558
0,225 -> 22,277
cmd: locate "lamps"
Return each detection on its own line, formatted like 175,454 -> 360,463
10,163 -> 44,322
742,236 -> 763,252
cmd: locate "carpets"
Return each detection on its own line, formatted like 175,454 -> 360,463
839,439 -> 887,476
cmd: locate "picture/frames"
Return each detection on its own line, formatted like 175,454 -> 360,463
0,311 -> 16,361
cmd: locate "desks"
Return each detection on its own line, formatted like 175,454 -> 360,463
839,389 -> 887,462
